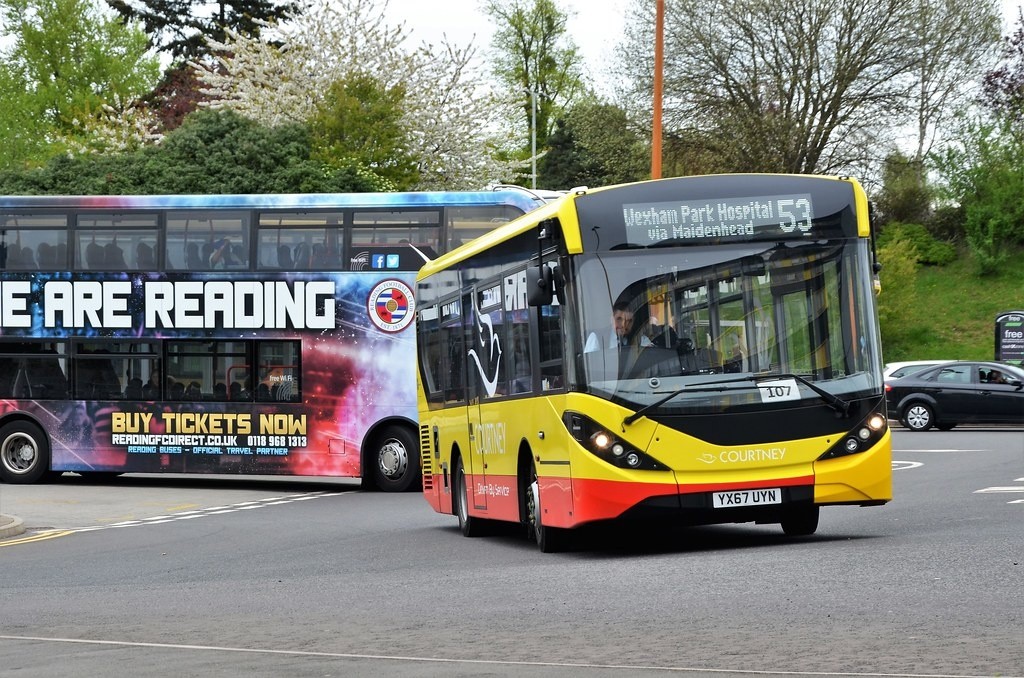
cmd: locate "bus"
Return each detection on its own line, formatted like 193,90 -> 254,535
413,172 -> 893,553
0,184 -> 570,492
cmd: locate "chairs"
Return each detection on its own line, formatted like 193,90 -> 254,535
0,243 -> 340,271
979,370 -> 986,383
90,378 -> 277,402
424,316 -> 561,392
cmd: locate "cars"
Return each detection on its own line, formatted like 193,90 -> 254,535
880,360 -> 1024,433
883,359 -> 1018,384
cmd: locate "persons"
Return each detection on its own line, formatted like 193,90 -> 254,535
209,239 -> 244,269
987,370 -> 1007,384
583,302 -> 655,354
127,371 -> 291,403
